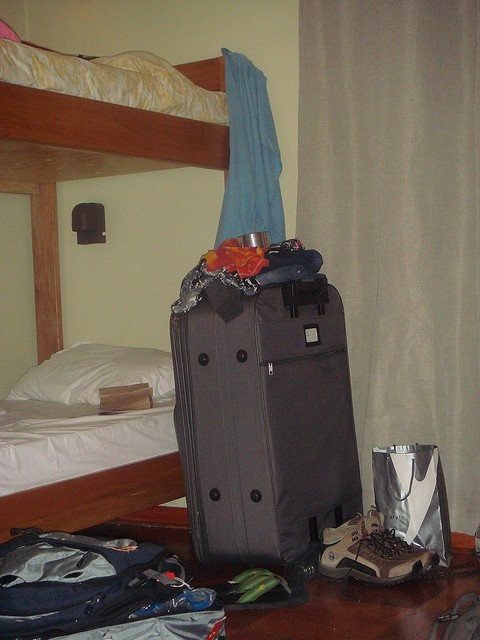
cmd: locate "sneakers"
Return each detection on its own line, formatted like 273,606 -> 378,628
364,507 -> 439,575
317,515 -> 423,586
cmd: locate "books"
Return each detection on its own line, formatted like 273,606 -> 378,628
97,383 -> 153,415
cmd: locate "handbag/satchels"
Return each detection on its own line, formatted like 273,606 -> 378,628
372,443 -> 452,567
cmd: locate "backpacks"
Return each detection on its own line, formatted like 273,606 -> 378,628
0,526 -> 227,640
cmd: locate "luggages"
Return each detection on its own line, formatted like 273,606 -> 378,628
169,232 -> 364,575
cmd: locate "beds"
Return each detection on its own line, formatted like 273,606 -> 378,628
0,34 -> 267,544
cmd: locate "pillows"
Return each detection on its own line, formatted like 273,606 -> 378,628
7,342 -> 176,404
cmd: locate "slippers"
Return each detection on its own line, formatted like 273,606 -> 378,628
214,576 -> 309,611
196,569 -> 303,596
